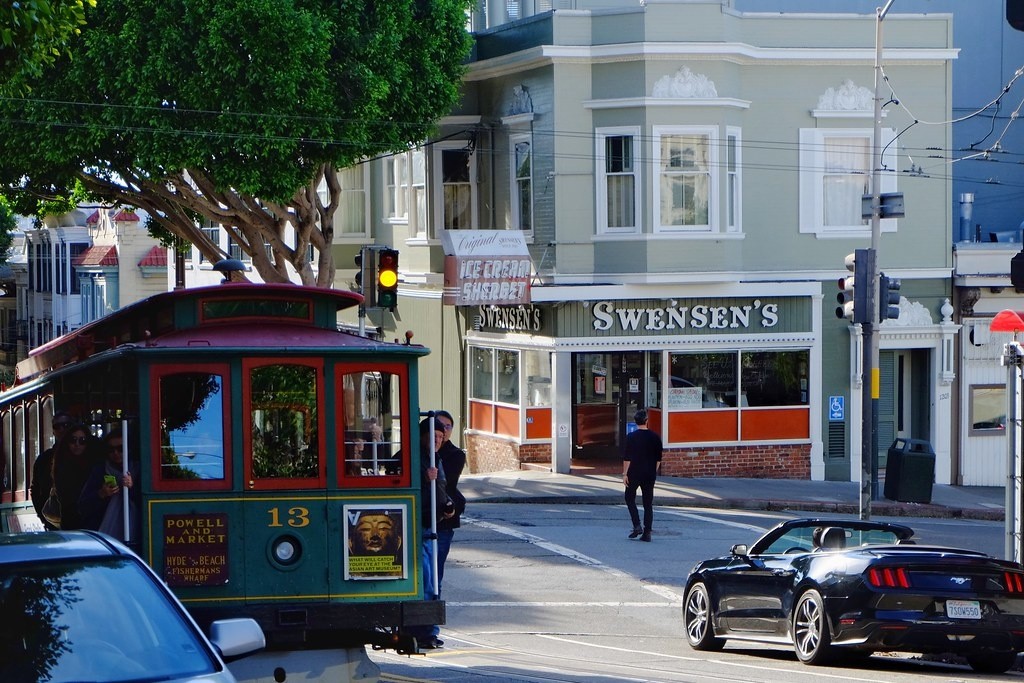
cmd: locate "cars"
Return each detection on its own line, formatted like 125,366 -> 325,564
0,529 -> 237,683
683,515 -> 1024,666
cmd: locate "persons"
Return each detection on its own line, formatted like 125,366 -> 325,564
621,409 -> 663,542
305,387 -> 387,476
383,410 -> 466,650
811,526 -> 827,548
26,412 -> 143,551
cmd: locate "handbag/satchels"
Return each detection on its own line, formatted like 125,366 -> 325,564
41,452 -> 61,529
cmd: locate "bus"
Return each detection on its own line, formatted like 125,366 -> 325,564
0,257 -> 447,653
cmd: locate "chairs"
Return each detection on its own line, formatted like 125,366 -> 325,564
821,526 -> 846,552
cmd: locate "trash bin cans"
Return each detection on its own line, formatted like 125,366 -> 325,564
884,438 -> 936,503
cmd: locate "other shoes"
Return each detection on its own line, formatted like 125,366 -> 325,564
418,636 -> 443,649
640,532 -> 652,542
629,526 -> 643,538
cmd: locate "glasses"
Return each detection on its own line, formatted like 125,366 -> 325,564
53,422 -> 73,430
442,424 -> 453,431
105,444 -> 122,453
68,436 -> 87,445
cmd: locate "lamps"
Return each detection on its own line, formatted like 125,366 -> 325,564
580,300 -> 589,307
670,298 -> 677,306
491,304 -> 499,311
519,304 -> 525,311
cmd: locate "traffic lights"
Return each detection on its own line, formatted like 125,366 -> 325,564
835,276 -> 854,323
880,275 -> 901,323
377,249 -> 399,308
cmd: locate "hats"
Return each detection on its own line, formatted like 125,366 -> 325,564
420,418 -> 444,434
